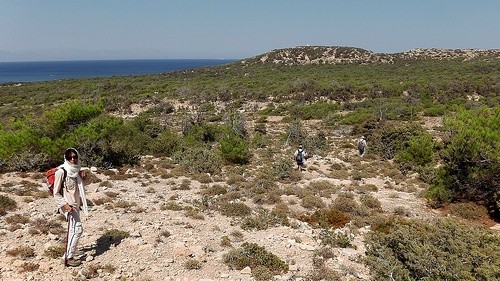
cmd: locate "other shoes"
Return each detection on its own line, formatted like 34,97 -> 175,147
60,257 -> 81,266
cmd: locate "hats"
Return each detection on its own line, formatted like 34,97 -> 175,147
299,145 -> 302,147
361,136 -> 366,139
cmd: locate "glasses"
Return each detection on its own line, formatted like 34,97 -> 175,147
66,156 -> 78,160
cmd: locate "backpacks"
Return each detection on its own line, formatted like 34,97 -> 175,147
359,140 -> 364,150
45,167 -> 67,197
296,149 -> 304,161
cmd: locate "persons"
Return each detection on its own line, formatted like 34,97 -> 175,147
292,144 -> 308,174
51,148 -> 84,267
356,135 -> 369,162
